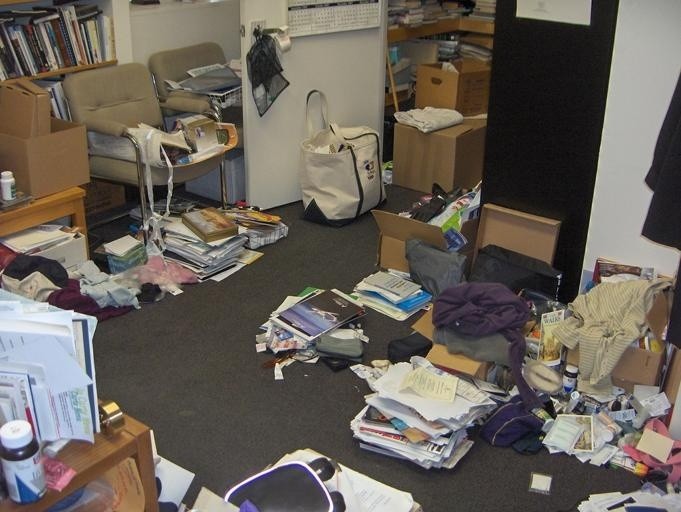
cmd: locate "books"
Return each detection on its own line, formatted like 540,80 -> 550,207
384,0 -> 499,97
349,356 -> 508,470
120,111 -> 229,171
165,55 -> 242,108
353,269 -> 432,322
1,288 -> 243,511
256,284 -> 366,364
264,447 -> 423,512
0,1 -> 116,127
518,259 -> 680,512
0,192 -> 292,284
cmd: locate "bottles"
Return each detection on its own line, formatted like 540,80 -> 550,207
1,169 -> 18,200
562,364 -> 579,394
600,424 -> 619,442
0,419 -> 47,506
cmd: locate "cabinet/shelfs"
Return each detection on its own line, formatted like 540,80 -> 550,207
384,16 -> 494,107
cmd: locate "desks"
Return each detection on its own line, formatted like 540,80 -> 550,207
0,399 -> 162,511
0,186 -> 91,263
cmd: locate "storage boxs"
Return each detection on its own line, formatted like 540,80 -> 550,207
1,117 -> 90,201
565,257 -> 676,395
411,55 -> 489,116
389,116 -> 485,196
412,306 -> 536,384
28,225 -> 88,274
184,153 -> 245,205
371,207 -> 477,278
78,179 -> 127,216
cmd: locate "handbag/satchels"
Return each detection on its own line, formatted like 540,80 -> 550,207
298,87 -> 389,226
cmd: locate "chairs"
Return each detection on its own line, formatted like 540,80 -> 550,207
59,60 -> 227,236
147,42 -> 243,209
